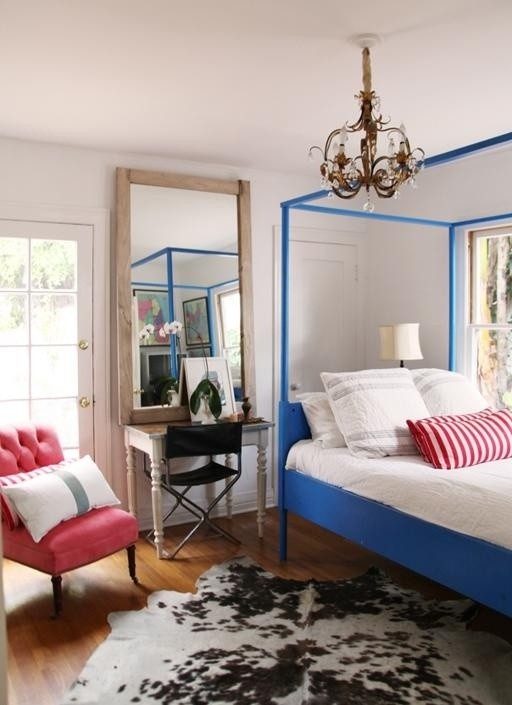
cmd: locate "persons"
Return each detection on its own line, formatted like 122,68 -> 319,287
203,372 -> 225,407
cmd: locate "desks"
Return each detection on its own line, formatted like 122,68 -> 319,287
123,420 -> 275,560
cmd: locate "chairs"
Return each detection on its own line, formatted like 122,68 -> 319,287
141,422 -> 243,560
279,131 -> 512,617
0,425 -> 145,617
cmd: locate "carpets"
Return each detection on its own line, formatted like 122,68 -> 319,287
61,552 -> 512,705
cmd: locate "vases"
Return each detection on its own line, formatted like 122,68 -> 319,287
201,394 -> 217,423
167,389 -> 181,407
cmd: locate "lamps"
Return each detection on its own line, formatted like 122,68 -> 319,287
306,32 -> 425,213
378,323 -> 424,367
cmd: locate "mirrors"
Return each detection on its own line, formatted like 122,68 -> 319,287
115,166 -> 257,424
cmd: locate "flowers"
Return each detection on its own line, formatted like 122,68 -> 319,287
159,321 -> 226,418
139,324 -> 184,393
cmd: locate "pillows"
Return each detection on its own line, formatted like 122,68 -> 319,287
300,366 -> 512,469
0,454 -> 121,544
0,457 -> 81,532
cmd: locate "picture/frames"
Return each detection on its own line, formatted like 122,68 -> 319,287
186,347 -> 212,357
132,289 -> 170,348
185,357 -> 237,421
182,296 -> 212,345
